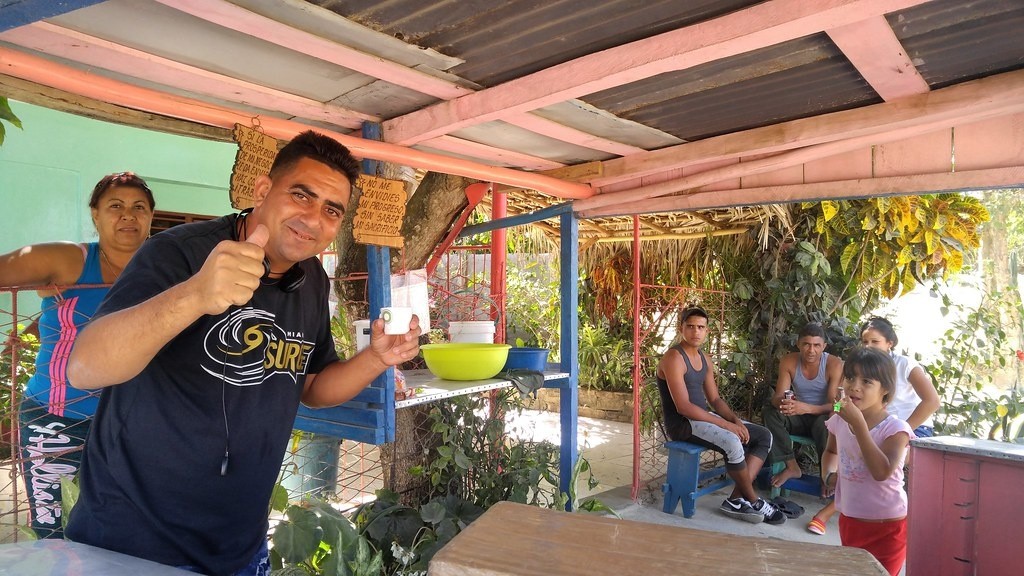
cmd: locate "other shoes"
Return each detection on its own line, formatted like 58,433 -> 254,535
807,516 -> 826,534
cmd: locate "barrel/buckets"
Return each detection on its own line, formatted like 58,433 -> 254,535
448,321 -> 496,344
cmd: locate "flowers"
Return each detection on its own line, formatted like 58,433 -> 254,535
264,385 -> 624,576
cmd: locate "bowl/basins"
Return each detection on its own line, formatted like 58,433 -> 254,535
501,348 -> 550,373
420,343 -> 512,381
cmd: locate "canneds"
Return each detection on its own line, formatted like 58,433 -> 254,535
784,389 -> 794,409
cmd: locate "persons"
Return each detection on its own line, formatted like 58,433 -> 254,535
655,304 -> 788,525
806,316 -> 941,535
0,171 -> 156,540
63,130 -> 422,576
821,346 -> 917,576
761,323 -> 843,499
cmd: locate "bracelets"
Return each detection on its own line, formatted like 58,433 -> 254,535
826,472 -> 833,486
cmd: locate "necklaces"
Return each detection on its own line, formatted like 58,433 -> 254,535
99,248 -> 120,280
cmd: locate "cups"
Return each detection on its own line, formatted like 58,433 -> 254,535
379,307 -> 412,335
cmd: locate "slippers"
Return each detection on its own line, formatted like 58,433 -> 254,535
769,496 -> 805,518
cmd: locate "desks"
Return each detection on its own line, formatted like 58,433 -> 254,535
428,502 -> 891,576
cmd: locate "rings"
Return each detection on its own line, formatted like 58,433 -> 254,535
832,401 -> 846,412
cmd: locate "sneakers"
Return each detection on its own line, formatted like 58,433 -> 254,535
750,498 -> 787,524
720,497 -> 764,523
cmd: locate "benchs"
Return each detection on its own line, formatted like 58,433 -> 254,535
661,435 -> 835,520
